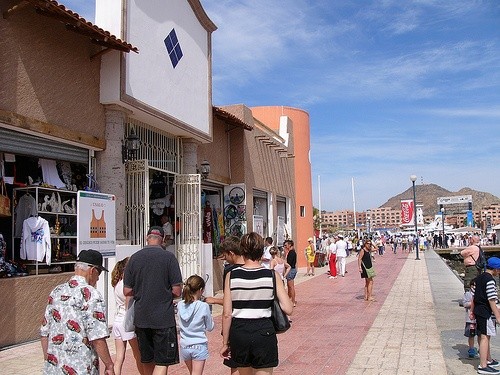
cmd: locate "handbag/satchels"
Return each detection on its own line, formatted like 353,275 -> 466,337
361,260 -> 376,278
270,269 -> 291,334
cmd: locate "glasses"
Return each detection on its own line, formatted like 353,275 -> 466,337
366,243 -> 372,244
88,265 -> 102,275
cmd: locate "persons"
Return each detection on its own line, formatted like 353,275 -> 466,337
303,237 -> 316,277
462,276 -> 481,359
110,256 -> 151,375
122,226 -> 186,374
221,231 -> 294,375
39,249 -> 115,375
160,216 -> 175,250
177,274 -> 215,375
470,257 -> 500,375
358,239 -> 379,301
458,234 -> 485,308
203,235 -> 246,374
316,227 -> 497,280
261,236 -> 298,307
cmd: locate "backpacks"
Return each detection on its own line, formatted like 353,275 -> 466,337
470,245 -> 486,270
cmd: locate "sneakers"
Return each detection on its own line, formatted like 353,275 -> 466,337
468,348 -> 475,359
486,357 -> 500,367
477,364 -> 500,375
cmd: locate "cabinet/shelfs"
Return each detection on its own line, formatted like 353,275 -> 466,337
11,187 -> 77,277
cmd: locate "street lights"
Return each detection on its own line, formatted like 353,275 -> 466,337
410,174 -> 421,260
441,208 -> 445,248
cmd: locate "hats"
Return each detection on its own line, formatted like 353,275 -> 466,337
147,226 -> 164,238
75,249 -> 109,272
487,257 -> 500,269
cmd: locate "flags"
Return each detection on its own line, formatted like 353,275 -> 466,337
400,197 -> 415,227
